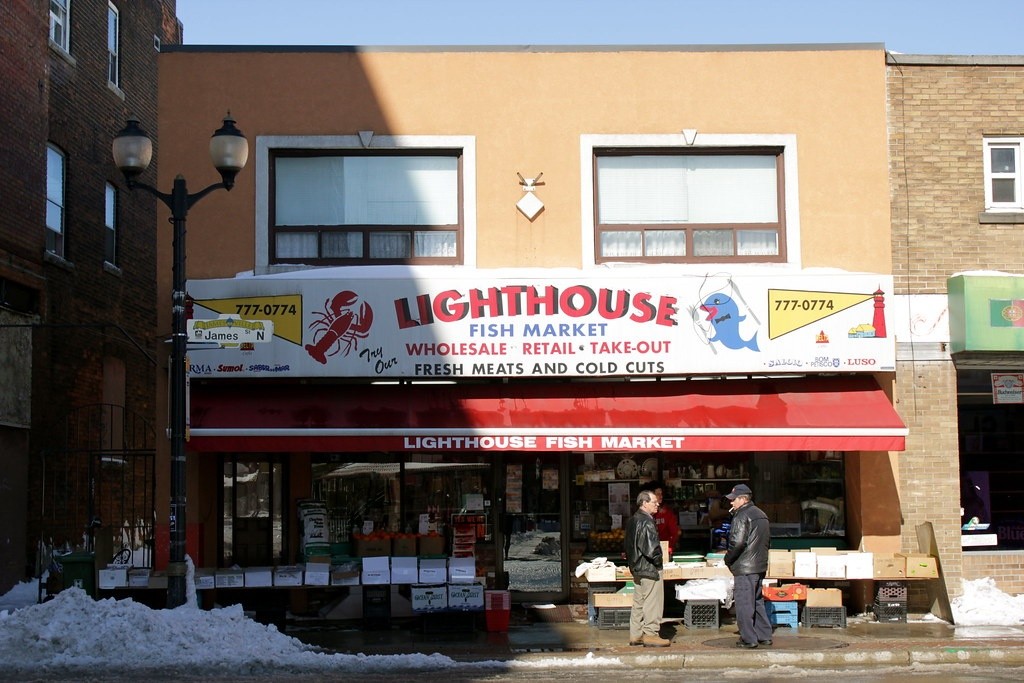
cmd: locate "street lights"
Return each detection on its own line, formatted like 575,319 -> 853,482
113,108 -> 249,608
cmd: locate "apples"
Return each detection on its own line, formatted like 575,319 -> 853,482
352,530 -> 440,540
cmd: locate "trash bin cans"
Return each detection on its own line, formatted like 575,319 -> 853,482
53,552 -> 96,596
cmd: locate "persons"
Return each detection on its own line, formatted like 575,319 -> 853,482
623,490 -> 671,648
642,480 -> 681,556
724,484 -> 772,648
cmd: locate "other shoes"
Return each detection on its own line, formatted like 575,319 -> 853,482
736,639 -> 758,649
758,639 -> 772,646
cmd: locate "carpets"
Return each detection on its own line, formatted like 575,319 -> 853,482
527,605 -> 574,623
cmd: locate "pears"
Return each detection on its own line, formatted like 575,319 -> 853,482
589,529 -> 626,551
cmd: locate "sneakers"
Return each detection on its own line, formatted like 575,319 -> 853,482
630,639 -> 643,647
643,634 -> 670,647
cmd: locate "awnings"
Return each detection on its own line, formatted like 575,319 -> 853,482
188,380 -> 908,452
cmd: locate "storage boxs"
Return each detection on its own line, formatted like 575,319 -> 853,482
149,571 -> 168,588
575,545 -> 939,629
484,590 -> 511,632
98,564 -> 134,589
128,569 -> 150,586
191,537 -> 486,612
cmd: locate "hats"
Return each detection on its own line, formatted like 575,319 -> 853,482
726,484 -> 751,500
